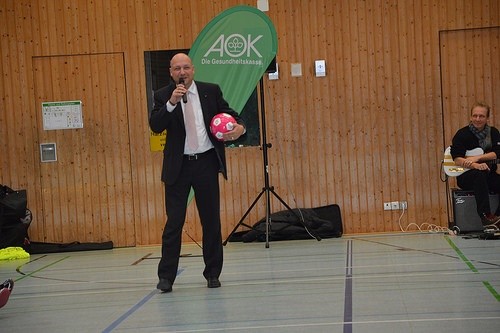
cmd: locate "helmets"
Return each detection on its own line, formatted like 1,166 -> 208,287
0,278 -> 14,308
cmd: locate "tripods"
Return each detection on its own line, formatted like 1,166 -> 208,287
222,76 -> 321,248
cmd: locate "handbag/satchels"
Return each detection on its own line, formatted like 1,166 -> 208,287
0,182 -> 28,227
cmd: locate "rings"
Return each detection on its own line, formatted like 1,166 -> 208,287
232,137 -> 233,139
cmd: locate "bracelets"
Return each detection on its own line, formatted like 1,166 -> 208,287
169,101 -> 175,106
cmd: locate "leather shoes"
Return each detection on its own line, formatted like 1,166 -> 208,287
207,276 -> 221,288
156,278 -> 172,292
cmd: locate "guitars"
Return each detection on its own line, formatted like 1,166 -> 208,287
442,145 -> 500,177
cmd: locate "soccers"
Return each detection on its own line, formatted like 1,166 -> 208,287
209,112 -> 237,142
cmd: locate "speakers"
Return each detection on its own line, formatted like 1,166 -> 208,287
451,189 -> 500,232
265,55 -> 276,73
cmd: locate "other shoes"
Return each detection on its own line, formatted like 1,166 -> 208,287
480,212 -> 500,228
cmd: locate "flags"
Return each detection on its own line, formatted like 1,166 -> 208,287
187,5 -> 277,202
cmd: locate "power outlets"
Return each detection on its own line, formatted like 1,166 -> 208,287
400,202 -> 407,210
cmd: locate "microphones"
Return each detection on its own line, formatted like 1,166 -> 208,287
178,78 -> 188,104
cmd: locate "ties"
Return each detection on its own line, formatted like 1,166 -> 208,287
183,91 -> 199,156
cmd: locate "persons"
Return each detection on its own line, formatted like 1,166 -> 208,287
450,102 -> 500,233
149,54 -> 248,293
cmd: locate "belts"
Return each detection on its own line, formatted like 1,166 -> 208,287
183,147 -> 215,158
